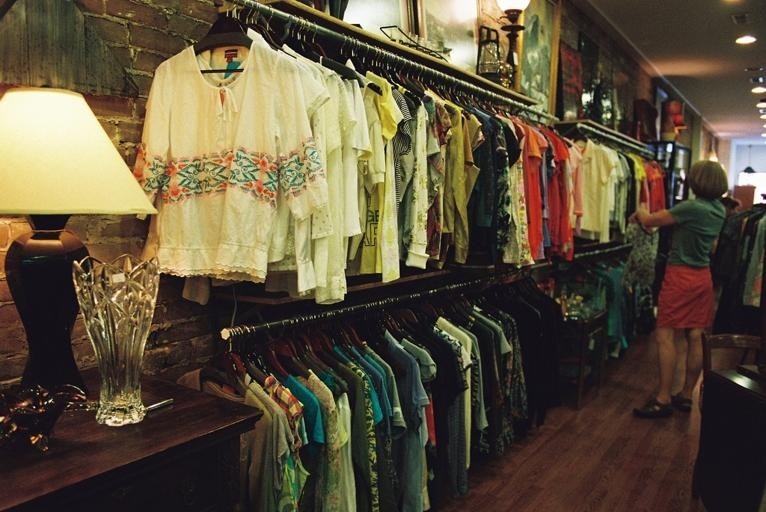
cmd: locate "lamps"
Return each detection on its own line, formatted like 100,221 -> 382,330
731,13 -> 756,46
750,77 -> 765,95
0,85 -> 160,399
495,0 -> 531,94
756,98 -> 766,113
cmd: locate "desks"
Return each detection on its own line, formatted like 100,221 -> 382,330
0,364 -> 264,511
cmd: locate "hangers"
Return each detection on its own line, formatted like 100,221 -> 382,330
367,275 -> 485,332
201,305 -> 363,379
215,0 -> 508,117
542,120 -> 664,158
486,260 -> 540,301
573,244 -> 623,268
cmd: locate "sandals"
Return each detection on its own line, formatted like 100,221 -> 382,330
632,398 -> 675,418
672,392 -> 692,413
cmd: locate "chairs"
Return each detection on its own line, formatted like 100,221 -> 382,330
702,331 -> 766,393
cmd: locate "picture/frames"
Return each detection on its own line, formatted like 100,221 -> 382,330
518,1 -> 557,113
560,40 -> 584,121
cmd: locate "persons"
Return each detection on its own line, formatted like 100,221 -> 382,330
628,160 -> 729,419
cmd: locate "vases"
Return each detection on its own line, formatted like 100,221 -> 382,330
66,253 -> 160,427
